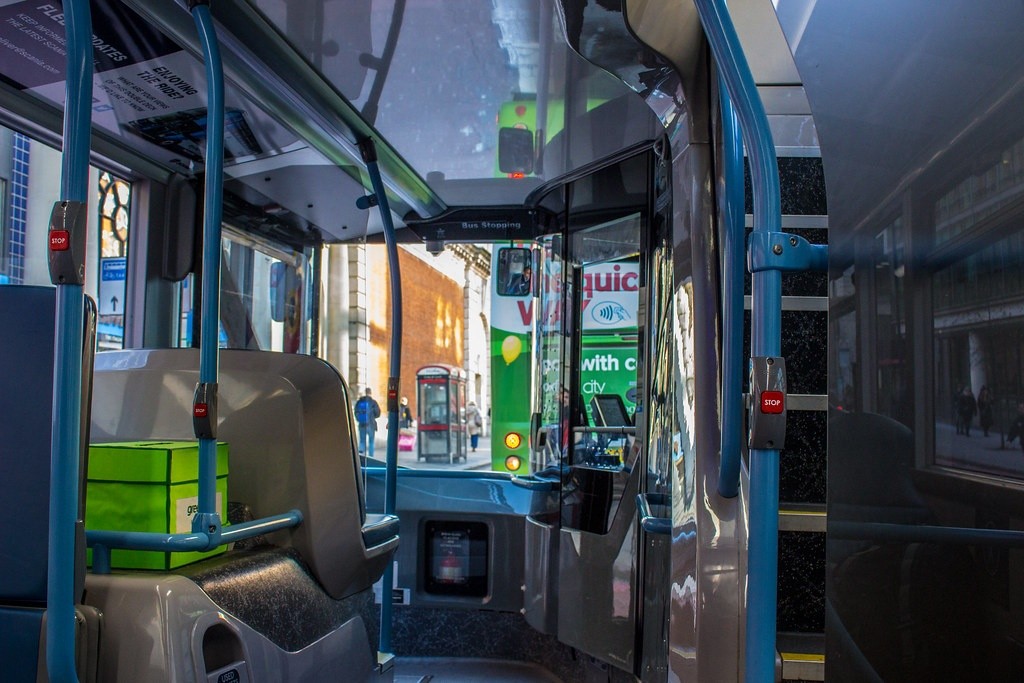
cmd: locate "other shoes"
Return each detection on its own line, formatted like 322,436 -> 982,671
472,448 -> 476,452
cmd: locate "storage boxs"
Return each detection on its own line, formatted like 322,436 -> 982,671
84,439 -> 230,573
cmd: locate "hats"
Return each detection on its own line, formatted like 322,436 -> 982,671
400,397 -> 408,406
468,401 -> 476,405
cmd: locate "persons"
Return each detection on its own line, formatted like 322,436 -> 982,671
463,402 -> 481,452
354,387 -> 380,456
1006,400 -> 1024,456
399,397 -> 413,430
953,385 -> 994,437
506,267 -> 530,295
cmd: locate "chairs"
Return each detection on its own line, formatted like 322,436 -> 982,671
83,346 -> 401,618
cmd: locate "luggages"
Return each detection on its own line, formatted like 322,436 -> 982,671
399,426 -> 418,451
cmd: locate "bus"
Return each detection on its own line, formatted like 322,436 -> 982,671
489,208 -> 640,475
494,96 -> 619,181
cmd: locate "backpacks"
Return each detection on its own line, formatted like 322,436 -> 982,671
357,401 -> 374,425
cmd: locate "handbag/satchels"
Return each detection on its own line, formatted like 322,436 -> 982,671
400,428 -> 416,436
475,413 -> 482,428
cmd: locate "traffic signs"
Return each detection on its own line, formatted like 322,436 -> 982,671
98,257 -> 127,317
